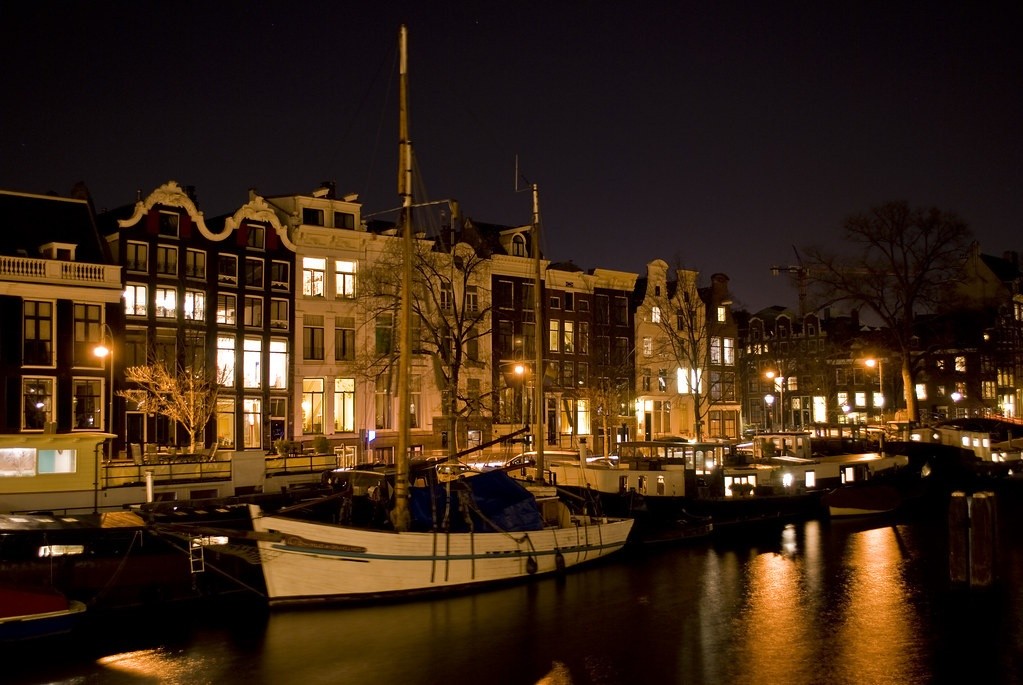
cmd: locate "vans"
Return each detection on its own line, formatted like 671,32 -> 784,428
496,450 -> 580,474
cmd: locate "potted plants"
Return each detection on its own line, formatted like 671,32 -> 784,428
273,437 -> 291,459
312,434 -> 332,453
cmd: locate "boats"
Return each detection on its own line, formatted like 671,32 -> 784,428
481,432 -> 910,513
0,513 -> 87,626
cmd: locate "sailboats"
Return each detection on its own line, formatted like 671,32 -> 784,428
249,27 -> 635,605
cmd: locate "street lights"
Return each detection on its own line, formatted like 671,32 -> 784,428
951,392 -> 962,428
30,386 -> 47,428
841,404 -> 851,432
94,323 -> 116,461
864,357 -> 884,450
765,370 -> 784,436
634,398 -> 641,443
513,347 -> 531,476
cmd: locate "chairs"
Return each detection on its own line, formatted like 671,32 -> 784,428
192,441 -> 204,462
130,442 -> 144,465
204,441 -> 219,462
143,441 -> 154,459
146,442 -> 161,464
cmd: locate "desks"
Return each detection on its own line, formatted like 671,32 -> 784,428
144,451 -> 199,463
535,496 -> 560,523
